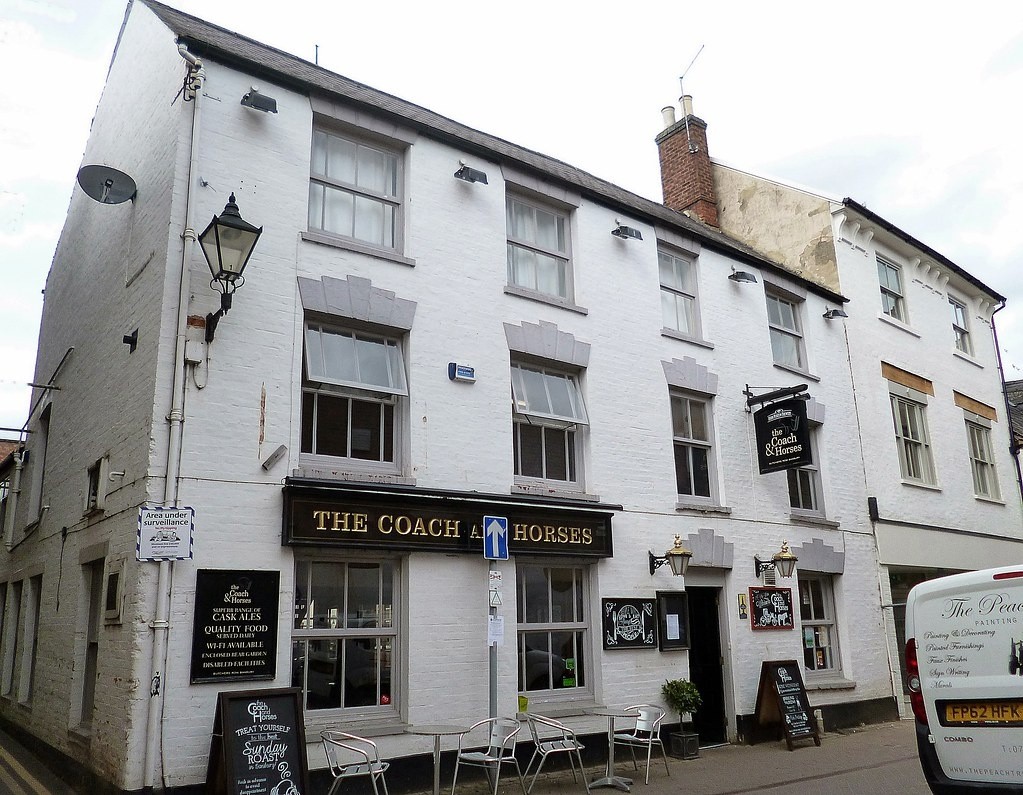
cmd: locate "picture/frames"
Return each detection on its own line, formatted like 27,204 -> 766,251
602,597 -> 658,650
657,590 -> 691,651
749,586 -> 794,630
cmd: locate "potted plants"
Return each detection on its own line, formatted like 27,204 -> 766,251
661,678 -> 702,760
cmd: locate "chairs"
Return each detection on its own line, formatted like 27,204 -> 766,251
451,716 -> 526,795
522,712 -> 590,795
319,730 -> 390,795
605,703 -> 670,785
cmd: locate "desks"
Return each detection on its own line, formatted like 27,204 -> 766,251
403,725 -> 470,795
583,709 -> 641,793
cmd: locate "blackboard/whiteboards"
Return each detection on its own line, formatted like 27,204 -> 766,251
754,660 -> 818,740
205,688 -> 307,795
750,402 -> 812,472
748,587 -> 795,630
191,570 -> 280,687
601,598 -> 657,650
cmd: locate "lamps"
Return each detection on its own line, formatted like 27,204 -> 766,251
453,158 -> 488,185
241,85 -> 277,114
648,535 -> 693,578
754,539 -> 799,578
610,218 -> 643,241
728,265 -> 758,283
822,305 -> 848,319
196,192 -> 263,342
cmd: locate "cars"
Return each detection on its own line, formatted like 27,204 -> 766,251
518,643 -> 569,691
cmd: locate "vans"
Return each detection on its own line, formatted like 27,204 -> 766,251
904,563 -> 1023,795
